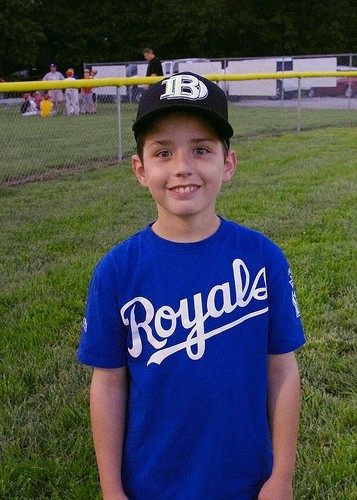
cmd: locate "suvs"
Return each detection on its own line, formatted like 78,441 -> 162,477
92,57 -> 210,105
219,57 -> 295,101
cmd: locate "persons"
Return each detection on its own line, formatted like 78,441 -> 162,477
76,71 -> 307,500
80,69 -> 94,114
41,64 -> 66,114
63,70 -> 80,116
33,91 -> 43,110
90,69 -> 97,113
21,93 -> 37,116
143,48 -> 164,91
68,68 -> 74,77
40,94 -> 57,117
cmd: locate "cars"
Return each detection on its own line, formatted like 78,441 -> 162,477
8,68 -> 46,79
308,65 -> 357,98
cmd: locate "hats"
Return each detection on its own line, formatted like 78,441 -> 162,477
66,70 -> 72,77
91,70 -> 97,74
84,69 -> 90,73
50,64 -> 56,67
24,93 -> 30,100
44,94 -> 50,100
132,72 -> 233,140
